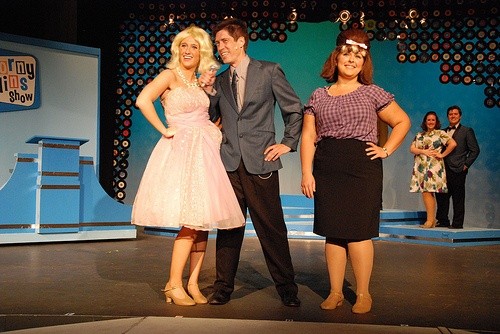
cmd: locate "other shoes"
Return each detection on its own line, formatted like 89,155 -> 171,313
282,295 -> 301,307
209,290 -> 231,304
436,222 -> 460,229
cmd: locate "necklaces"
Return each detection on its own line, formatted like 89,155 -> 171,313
177,69 -> 198,87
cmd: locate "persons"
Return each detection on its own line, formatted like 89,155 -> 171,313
136,25 -> 245,305
435,105 -> 480,229
409,111 -> 457,228
204,19 -> 304,306
300,30 -> 411,313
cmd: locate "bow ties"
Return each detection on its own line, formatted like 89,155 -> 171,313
448,125 -> 456,131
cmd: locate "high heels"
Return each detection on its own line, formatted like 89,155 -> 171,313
320,291 -> 344,310
186,280 -> 208,304
161,280 -> 196,305
419,220 -> 436,228
352,292 -> 372,314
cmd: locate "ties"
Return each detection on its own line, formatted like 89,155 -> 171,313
231,69 -> 239,108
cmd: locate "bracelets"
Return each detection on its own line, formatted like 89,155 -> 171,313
383,146 -> 389,158
